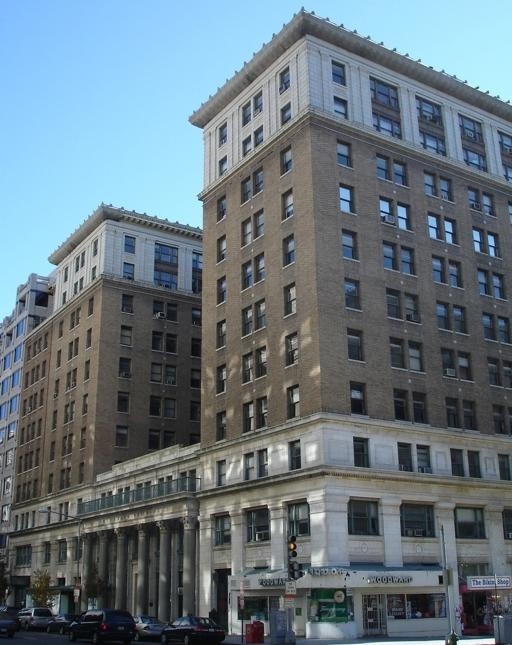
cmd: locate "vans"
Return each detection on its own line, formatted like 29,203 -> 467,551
15,607 -> 53,632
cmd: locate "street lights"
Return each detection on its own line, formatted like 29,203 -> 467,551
38,509 -> 81,617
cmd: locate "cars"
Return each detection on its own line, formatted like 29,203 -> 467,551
160,616 -> 225,645
46,614 -> 80,635
132,616 -> 169,642
68,609 -> 136,645
0,611 -> 19,638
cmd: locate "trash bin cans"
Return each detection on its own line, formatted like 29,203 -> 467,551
246,620 -> 264,645
493,615 -> 512,645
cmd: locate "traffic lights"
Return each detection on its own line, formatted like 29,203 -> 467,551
288,535 -> 298,558
